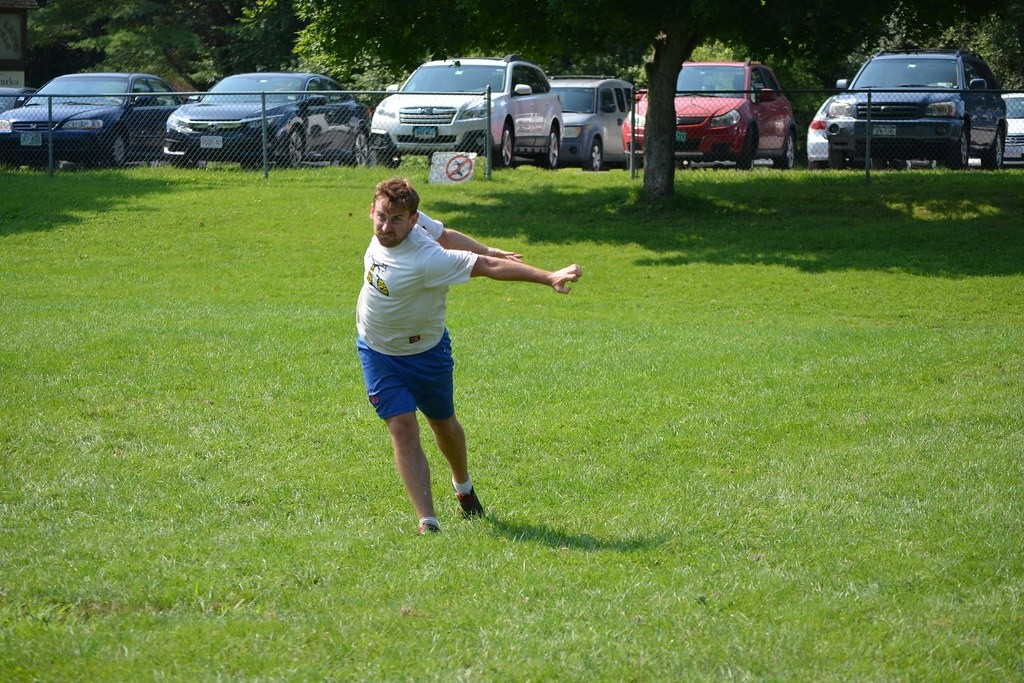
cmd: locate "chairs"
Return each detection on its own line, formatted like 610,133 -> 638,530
122,87 -> 142,104
482,77 -> 501,90
726,74 -> 745,99
271,87 -> 292,103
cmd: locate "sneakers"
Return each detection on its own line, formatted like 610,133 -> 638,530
417,522 -> 441,540
450,482 -> 485,521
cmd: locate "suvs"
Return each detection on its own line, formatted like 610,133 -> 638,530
622,57 -> 799,172
825,48 -> 1008,171
514,74 -> 644,172
368,54 -> 564,172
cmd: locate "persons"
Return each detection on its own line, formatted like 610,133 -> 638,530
354,178 -> 581,537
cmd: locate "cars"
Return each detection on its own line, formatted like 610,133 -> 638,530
0,86 -> 39,114
0,73 -> 208,169
807,94 -> 936,170
161,72 -> 372,169
1000,93 -> 1024,162
